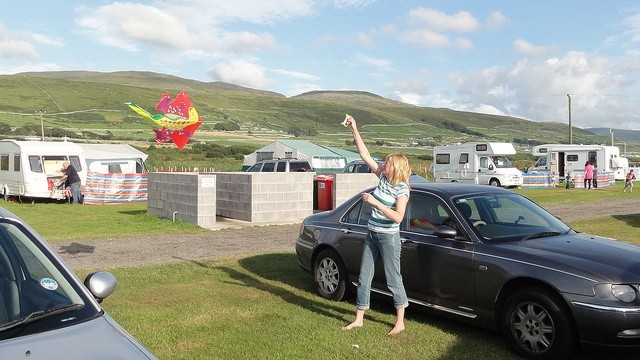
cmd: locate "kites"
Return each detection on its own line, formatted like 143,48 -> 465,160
124,90 -> 202,150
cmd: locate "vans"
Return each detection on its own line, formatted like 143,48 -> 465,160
0,139 -> 88,201
614,157 -> 629,181
433,141 -> 523,188
527,144 -> 624,187
245,157 -> 314,172
72,143 -> 149,173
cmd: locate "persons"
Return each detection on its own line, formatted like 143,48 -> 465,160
565,172 -> 572,188
59,162 -> 81,203
583,161 -> 593,190
524,165 -> 528,172
624,169 -> 636,192
592,164 -> 597,188
550,172 -> 556,187
341,113 -> 410,336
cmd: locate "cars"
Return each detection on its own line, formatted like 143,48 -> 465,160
340,160 -> 431,182
1,205 -> 162,360
295,182 -> 640,358
